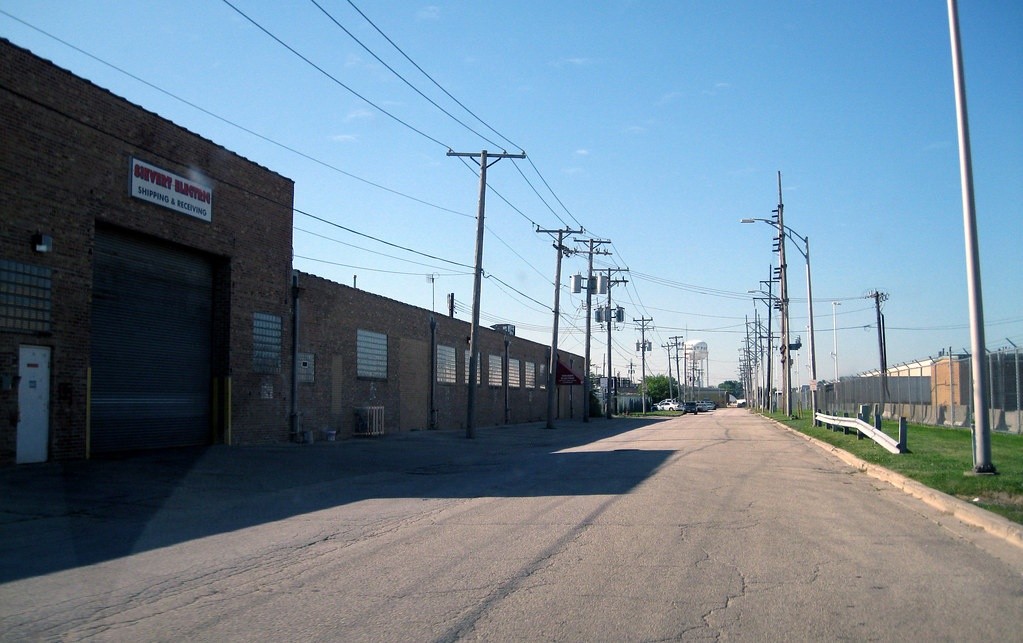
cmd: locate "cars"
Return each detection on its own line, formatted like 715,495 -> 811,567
706,401 -> 716,410
656,401 -> 682,410
682,401 -> 698,415
697,402 -> 707,412
653,398 -> 676,405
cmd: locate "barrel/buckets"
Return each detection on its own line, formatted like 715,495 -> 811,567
327,431 -> 337,442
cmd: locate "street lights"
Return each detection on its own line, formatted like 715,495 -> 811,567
831,301 -> 841,382
805,325 -> 811,383
738,218 -> 818,429
746,289 -> 786,415
